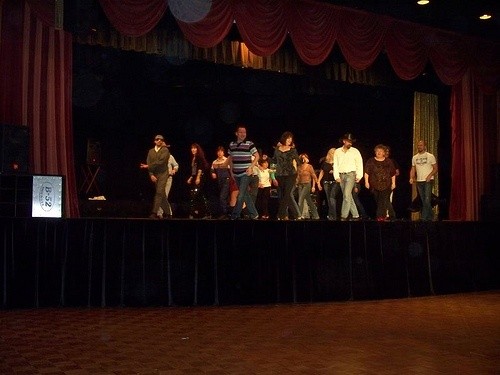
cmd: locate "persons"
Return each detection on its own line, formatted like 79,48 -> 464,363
409,140 -> 438,222
139,126 -> 400,222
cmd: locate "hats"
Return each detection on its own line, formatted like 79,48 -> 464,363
339,134 -> 356,145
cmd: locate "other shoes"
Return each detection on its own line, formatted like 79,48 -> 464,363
149,215 -> 438,221
